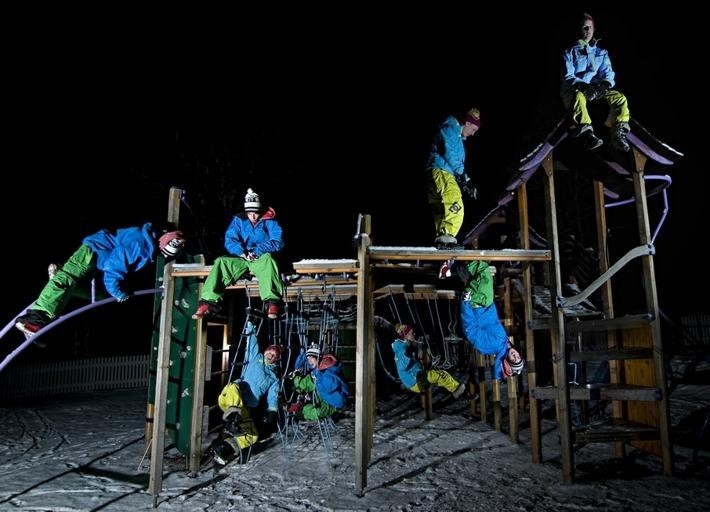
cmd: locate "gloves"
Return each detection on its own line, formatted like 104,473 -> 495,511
247,248 -> 259,261
246,314 -> 257,323
262,411 -> 278,426
462,178 -> 478,199
115,290 -> 130,303
462,290 -> 470,301
577,82 -> 597,101
594,83 -> 607,100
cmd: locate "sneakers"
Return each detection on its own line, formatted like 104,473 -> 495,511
439,260 -> 454,281
191,300 -> 214,319
453,382 -> 466,400
25,329 -> 48,348
211,445 -> 228,465
609,130 -> 630,152
434,234 -> 459,246
580,131 -> 603,151
224,421 -> 248,437
15,311 -> 46,333
460,393 -> 480,405
287,402 -> 302,413
266,300 -> 279,320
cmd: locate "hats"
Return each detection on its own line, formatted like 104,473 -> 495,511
152,218 -> 178,239
462,110 -> 481,129
398,325 -> 412,337
263,345 -> 282,361
244,193 -> 262,213
305,345 -> 321,359
505,362 -> 524,376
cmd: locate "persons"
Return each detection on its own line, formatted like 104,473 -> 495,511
557,11 -> 632,154
12,218 -> 186,351
425,107 -> 488,246
209,306 -> 284,467
189,186 -> 284,320
455,257 -> 522,384
390,322 -> 470,399
291,343 -> 348,420
